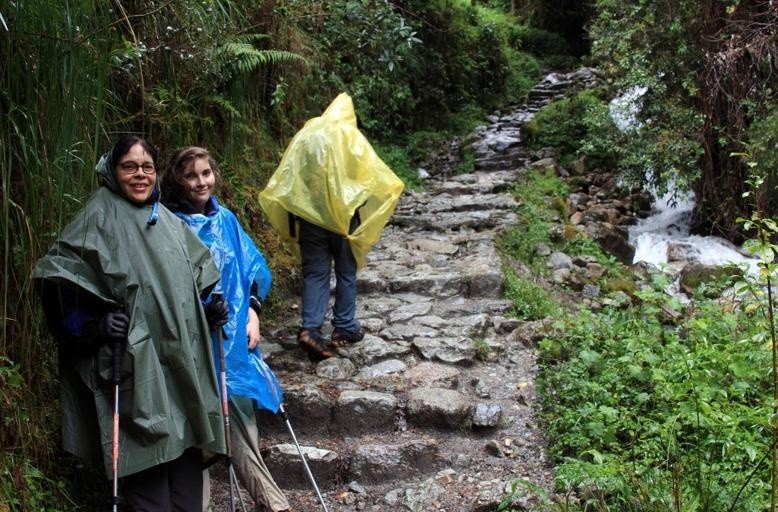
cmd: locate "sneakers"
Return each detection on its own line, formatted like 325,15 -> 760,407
330,327 -> 366,346
297,329 -> 335,360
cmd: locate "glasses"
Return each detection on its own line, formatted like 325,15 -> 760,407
117,161 -> 158,175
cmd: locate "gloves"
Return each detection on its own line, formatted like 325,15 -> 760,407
98,312 -> 129,345
202,298 -> 230,333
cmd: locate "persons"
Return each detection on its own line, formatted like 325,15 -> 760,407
258,92 -> 406,363
159,144 -> 295,511
29,133 -> 228,511
